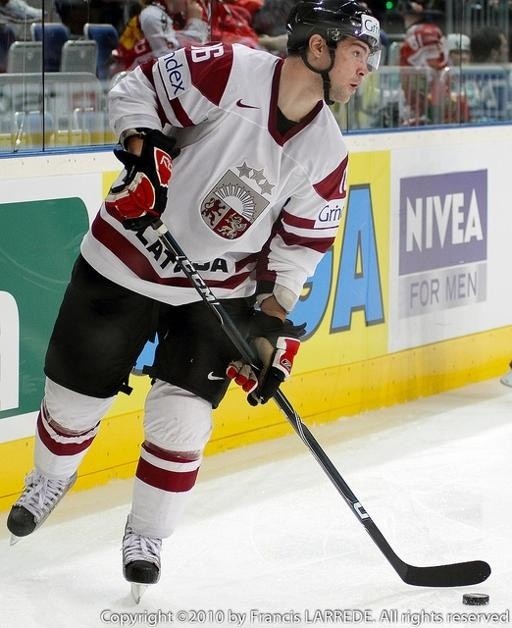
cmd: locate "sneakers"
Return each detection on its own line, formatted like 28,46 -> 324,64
122,517 -> 162,585
7,466 -> 78,537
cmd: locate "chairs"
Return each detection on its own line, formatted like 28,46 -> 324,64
6,20 -> 121,155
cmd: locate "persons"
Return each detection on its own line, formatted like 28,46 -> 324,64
8,2 -> 382,585
397,0 -> 510,126
110,0 -> 292,77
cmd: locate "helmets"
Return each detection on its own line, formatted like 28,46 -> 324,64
446,33 -> 471,53
285,0 -> 382,55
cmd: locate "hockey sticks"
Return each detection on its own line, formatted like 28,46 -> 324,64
150,215 -> 491,587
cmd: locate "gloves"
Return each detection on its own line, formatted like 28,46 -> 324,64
225,308 -> 309,406
104,129 -> 182,232
